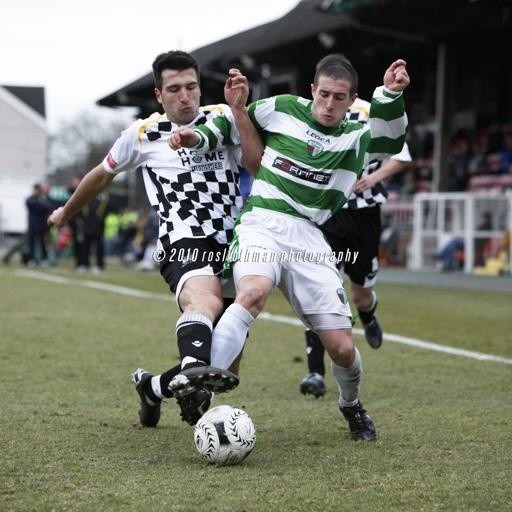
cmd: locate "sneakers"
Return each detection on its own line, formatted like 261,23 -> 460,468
301,374 -> 326,398
365,319 -> 382,349
339,399 -> 376,442
131,365 -> 238,428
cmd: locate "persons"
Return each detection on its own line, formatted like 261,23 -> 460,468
45,48 -> 264,432
208,53 -> 410,440
297,97 -> 413,397
23,98 -> 512,276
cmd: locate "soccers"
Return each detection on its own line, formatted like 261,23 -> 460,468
193,404 -> 258,466
